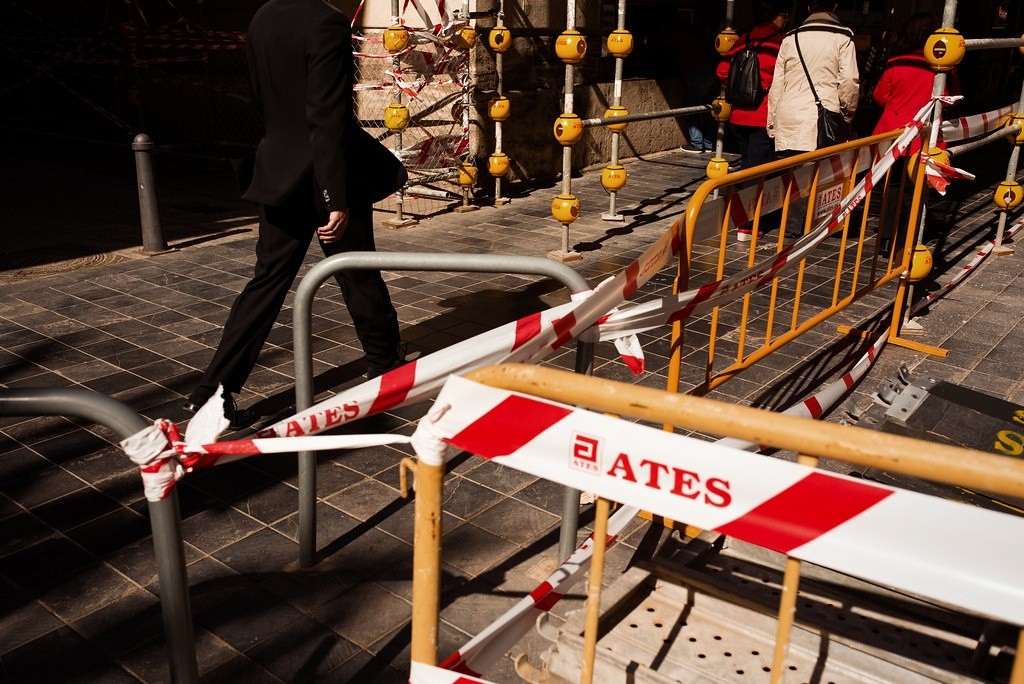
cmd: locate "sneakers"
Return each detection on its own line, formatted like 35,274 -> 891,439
737,225 -> 764,241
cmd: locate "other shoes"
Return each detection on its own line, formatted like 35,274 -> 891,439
880,238 -> 890,256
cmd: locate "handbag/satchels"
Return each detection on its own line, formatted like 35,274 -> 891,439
817,108 -> 858,149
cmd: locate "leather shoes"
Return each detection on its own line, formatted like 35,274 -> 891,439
367,359 -> 408,381
182,384 -> 255,429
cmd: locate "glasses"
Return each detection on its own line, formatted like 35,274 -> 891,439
779,10 -> 793,17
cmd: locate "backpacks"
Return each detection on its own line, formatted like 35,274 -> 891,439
726,31 -> 782,110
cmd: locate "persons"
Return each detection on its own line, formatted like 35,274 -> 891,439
182,1 -> 422,460
716,1 -> 961,262
